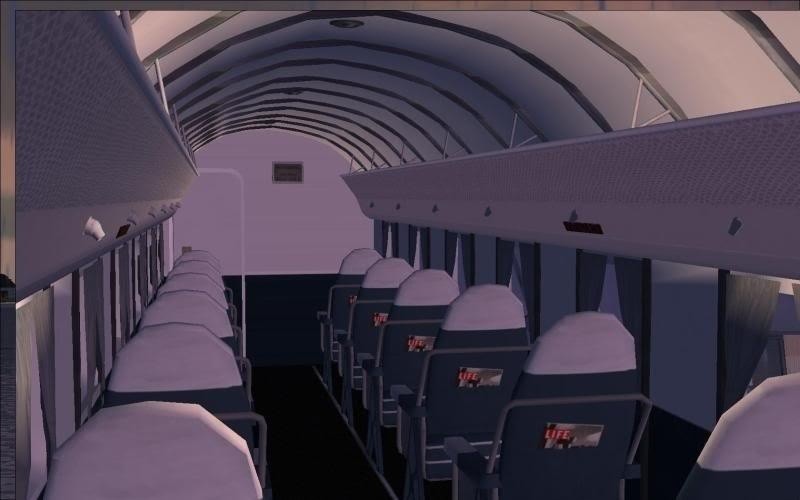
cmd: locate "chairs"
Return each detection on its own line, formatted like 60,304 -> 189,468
42,248 -> 270,500
679,370 -> 800,497
338,257 -> 417,424
317,248 -> 388,386
445,309 -> 652,499
392,282 -> 534,499
359,268 -> 461,456
